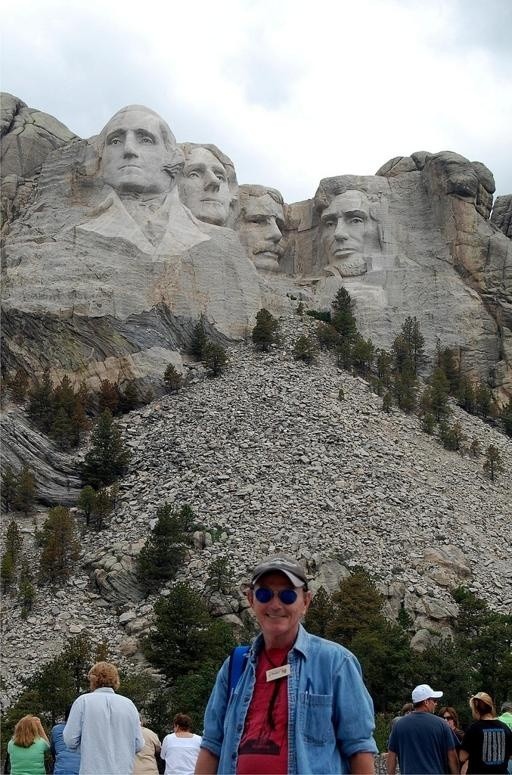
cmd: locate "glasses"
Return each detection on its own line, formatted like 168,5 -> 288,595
255,588 -> 297,604
442,717 -> 453,721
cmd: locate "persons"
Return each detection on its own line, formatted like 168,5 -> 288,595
389,703 -> 413,732
48,705 -> 84,775
385,683 -> 460,775
98,106 -> 382,285
135,712 -> 162,775
497,702 -> 512,775
159,713 -> 206,775
193,554 -> 379,775
63,660 -> 145,775
7,714 -> 51,775
438,707 -> 467,775
458,692 -> 511,775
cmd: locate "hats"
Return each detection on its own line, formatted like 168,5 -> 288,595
250,555 -> 308,591
412,684 -> 443,703
